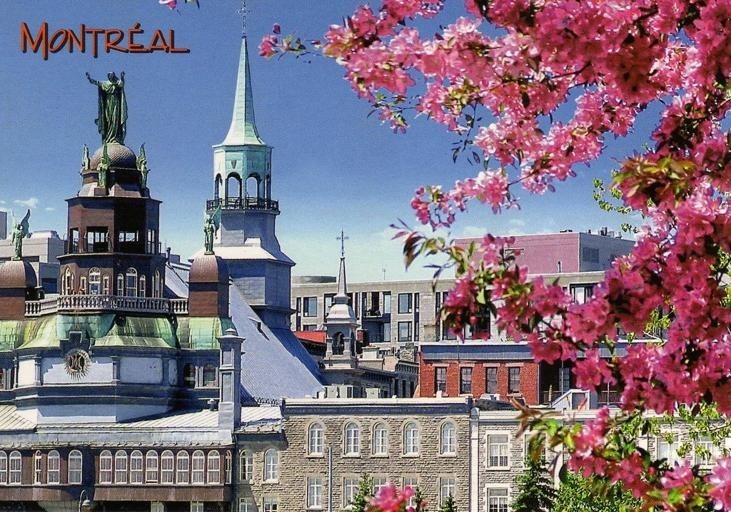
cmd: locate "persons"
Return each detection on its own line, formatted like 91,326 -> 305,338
9,223 -> 24,261
78,163 -> 85,184
85,70 -> 128,146
97,158 -> 107,188
141,161 -> 151,187
203,217 -> 217,255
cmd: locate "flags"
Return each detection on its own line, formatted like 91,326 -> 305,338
212,207 -> 222,230
